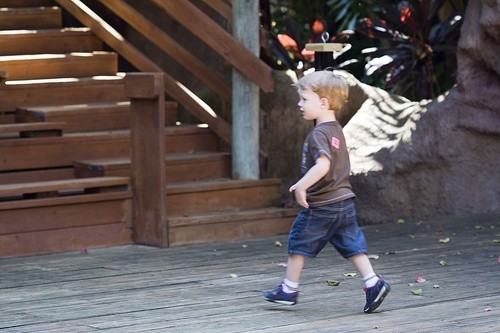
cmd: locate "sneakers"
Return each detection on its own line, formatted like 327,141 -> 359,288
262,281 -> 300,305
363,279 -> 391,313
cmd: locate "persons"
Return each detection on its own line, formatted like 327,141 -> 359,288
262,71 -> 392,315
